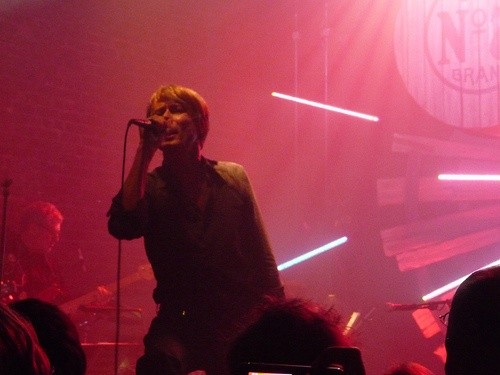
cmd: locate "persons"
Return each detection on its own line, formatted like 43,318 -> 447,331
0,200 -> 500,375
104,85 -> 283,375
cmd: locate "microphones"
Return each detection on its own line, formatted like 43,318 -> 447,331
383,303 -> 428,313
130,118 -> 159,131
